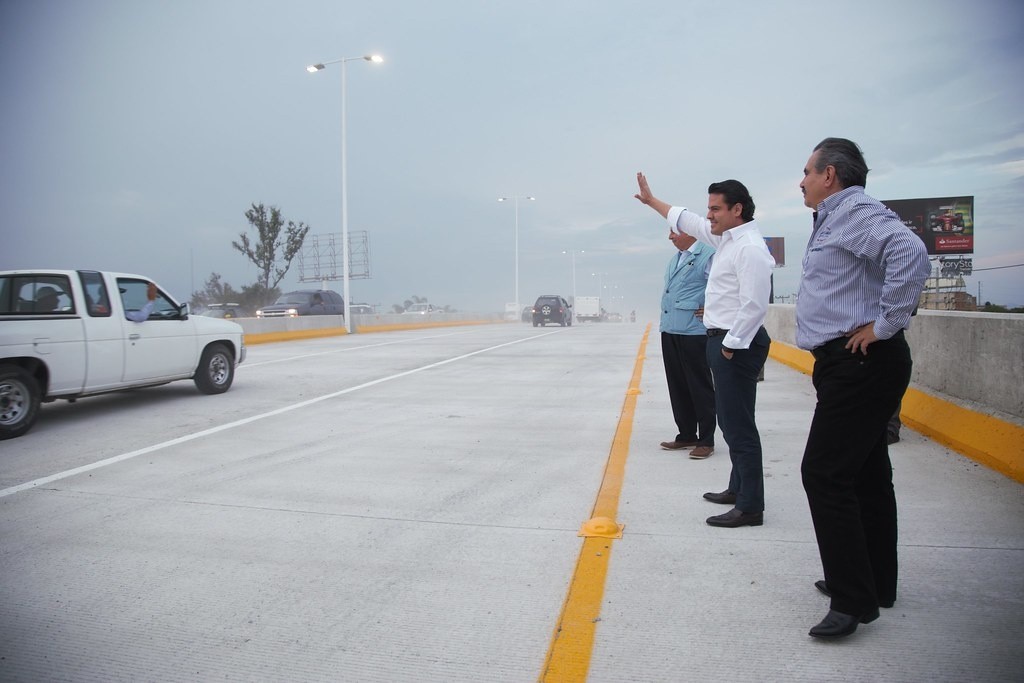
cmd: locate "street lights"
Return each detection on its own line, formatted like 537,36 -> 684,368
592,272 -> 623,313
498,196 -> 535,320
562,249 -> 584,318
306,54 -> 383,331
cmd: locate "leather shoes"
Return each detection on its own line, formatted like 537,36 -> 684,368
815,581 -> 894,608
809,608 -> 880,637
705,508 -> 763,527
660,442 -> 699,450
703,489 -> 737,504
689,446 -> 714,458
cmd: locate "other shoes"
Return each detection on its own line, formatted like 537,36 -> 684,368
886,436 -> 899,444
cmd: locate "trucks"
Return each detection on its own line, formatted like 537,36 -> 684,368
575,297 -> 603,323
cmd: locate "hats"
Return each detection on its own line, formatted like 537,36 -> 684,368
98,286 -> 127,294
37,286 -> 65,299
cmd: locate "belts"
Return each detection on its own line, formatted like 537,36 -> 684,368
810,328 -> 905,360
706,330 -> 729,336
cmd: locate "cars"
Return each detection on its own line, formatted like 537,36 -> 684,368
604,313 -> 622,322
199,303 -> 249,318
350,305 -> 376,314
401,303 -> 440,315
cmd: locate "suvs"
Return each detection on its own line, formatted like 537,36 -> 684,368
531,295 -> 573,328
257,290 -> 344,318
522,307 -> 533,323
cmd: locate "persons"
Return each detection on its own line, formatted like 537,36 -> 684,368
98,283 -> 158,322
886,402 -> 901,445
798,137 -> 930,640
635,173 -> 776,527
35,287 -> 66,309
659,227 -> 717,460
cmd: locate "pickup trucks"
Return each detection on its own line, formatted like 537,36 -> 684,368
0,270 -> 247,439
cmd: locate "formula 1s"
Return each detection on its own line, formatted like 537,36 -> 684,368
929,206 -> 964,232
